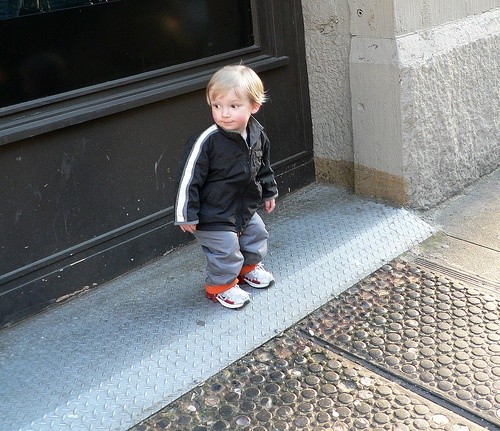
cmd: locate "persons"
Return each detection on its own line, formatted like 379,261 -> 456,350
174,64 -> 278,308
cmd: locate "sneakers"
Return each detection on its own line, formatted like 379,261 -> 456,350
241,262 -> 275,289
206,283 -> 250,309
